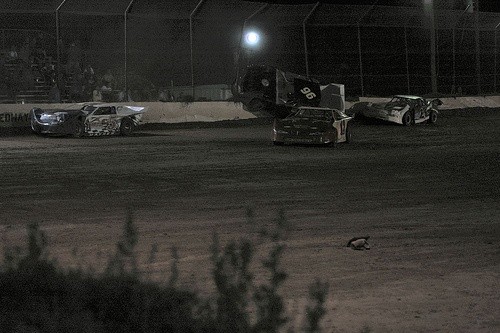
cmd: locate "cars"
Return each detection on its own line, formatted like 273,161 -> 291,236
30,101 -> 145,137
272,106 -> 353,145
376,95 -> 432,125
231,63 -> 321,118
